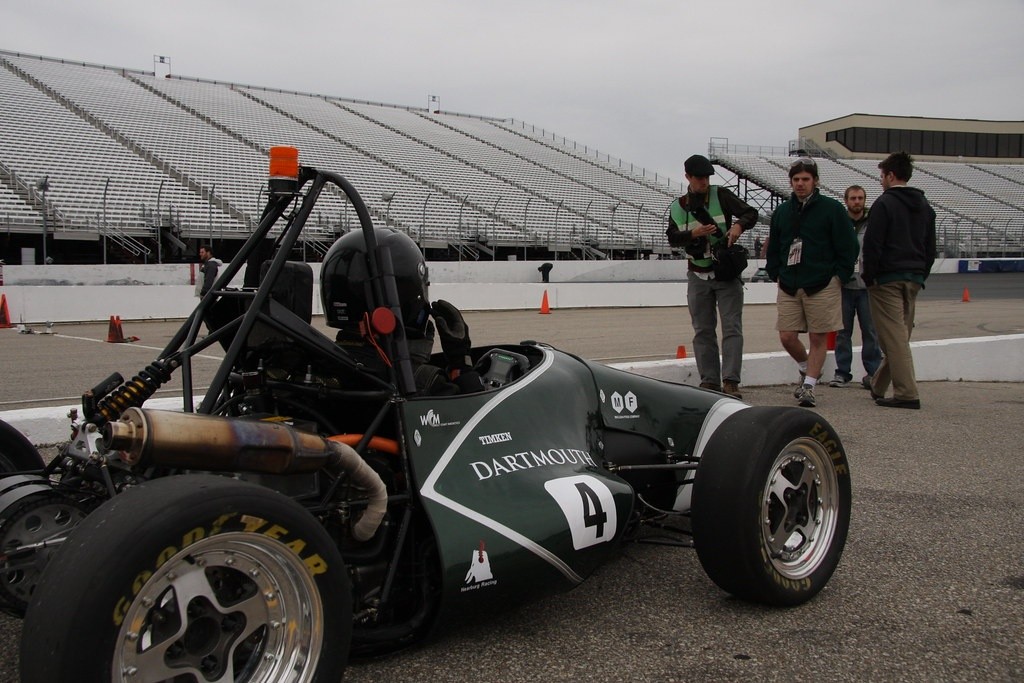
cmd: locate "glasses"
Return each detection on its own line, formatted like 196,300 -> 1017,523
790,158 -> 815,167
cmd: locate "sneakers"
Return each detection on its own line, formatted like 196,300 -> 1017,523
830,375 -> 849,388
798,383 -> 816,407
794,371 -> 807,398
700,382 -> 721,392
723,379 -> 742,399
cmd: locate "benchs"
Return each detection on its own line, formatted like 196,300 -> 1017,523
0,51 -> 1024,258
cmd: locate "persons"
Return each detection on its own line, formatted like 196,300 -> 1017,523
194,244 -> 222,337
755,152 -> 937,410
666,154 -> 759,399
319,226 -> 483,394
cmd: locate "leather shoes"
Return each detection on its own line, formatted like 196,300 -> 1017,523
875,395 -> 920,410
862,375 -> 884,400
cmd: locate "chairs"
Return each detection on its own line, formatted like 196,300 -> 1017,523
244,259 -> 397,438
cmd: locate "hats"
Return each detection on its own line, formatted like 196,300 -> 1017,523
684,155 -> 715,176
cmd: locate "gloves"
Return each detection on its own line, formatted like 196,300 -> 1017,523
431,300 -> 474,371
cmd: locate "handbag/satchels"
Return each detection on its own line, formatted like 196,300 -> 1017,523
727,244 -> 748,271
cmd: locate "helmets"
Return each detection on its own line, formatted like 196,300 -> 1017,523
320,226 -> 429,337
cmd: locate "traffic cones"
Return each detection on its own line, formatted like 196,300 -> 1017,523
825,331 -> 837,351
0,292 -> 17,329
538,290 -> 552,314
103,315 -> 125,343
116,316 -> 123,339
676,345 -> 688,358
960,287 -> 972,302
